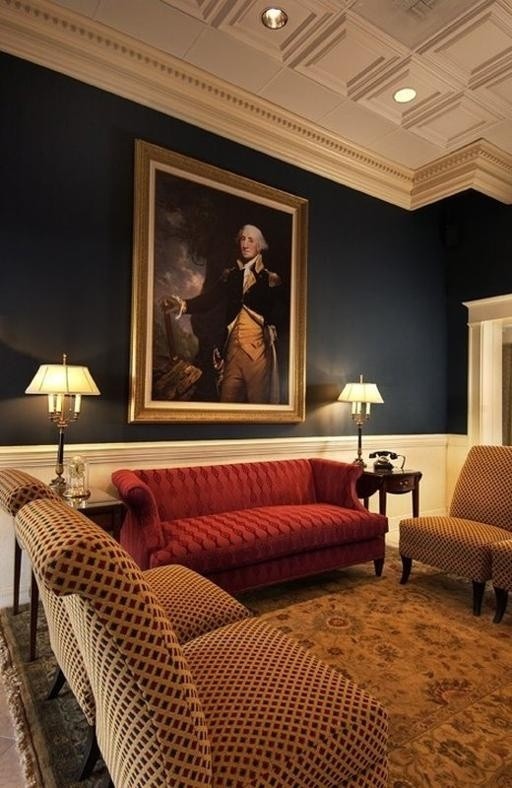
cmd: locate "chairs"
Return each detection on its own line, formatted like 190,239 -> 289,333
0,466 -> 251,780
399,445 -> 512,617
14,499 -> 387,788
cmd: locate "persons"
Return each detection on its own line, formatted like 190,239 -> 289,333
159,224 -> 286,404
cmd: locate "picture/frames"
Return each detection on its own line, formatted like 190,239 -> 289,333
129,137 -> 310,424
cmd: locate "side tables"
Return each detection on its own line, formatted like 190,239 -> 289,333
357,461 -> 421,518
14,492 -> 125,661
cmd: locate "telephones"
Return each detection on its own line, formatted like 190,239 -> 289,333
369,451 -> 398,471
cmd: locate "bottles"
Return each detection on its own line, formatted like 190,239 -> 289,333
66,456 -> 85,504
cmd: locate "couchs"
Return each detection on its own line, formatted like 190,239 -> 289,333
112,458 -> 388,597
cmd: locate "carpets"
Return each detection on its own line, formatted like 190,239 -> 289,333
0,540 -> 512,788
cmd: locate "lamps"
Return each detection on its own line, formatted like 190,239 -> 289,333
24,351 -> 99,490
335,374 -> 383,466
